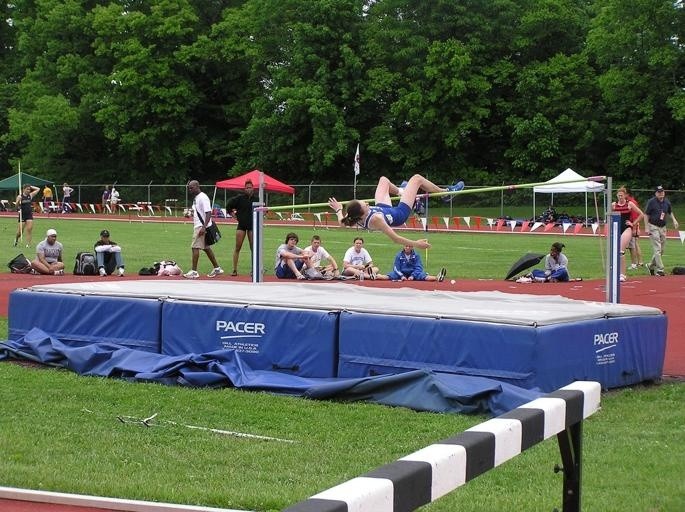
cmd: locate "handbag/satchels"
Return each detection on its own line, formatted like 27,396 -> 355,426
158,261 -> 182,276
204,221 -> 222,246
8,254 -> 31,273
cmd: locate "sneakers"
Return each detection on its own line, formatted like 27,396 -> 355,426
99,267 -> 107,277
368,267 -> 375,280
400,180 -> 408,188
620,263 -> 665,282
183,268 -> 237,279
32,269 -> 40,275
438,268 -> 446,282
118,268 -> 124,277
441,181 -> 464,202
54,270 -> 64,276
297,275 -> 306,279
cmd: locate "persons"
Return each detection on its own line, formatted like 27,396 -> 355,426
302,235 -> 340,278
31,228 -> 65,275
609,188 -> 644,282
562,211 -> 570,216
226,180 -> 263,276
327,174 -> 464,249
619,186 -> 643,270
518,242 -> 570,282
367,241 -> 447,282
342,237 -> 378,280
102,189 -> 110,213
111,188 -> 119,213
644,185 -> 678,276
274,232 -> 322,281
14,184 -> 40,249
61,183 -> 73,214
94,229 -> 125,277
413,196 -> 424,217
545,205 -> 555,214
42,183 -> 53,213
182,180 -> 225,278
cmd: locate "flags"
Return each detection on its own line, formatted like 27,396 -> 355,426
352,142 -> 361,176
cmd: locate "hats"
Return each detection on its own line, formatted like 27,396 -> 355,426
657,186 -> 664,192
47,229 -> 57,236
100,230 -> 109,236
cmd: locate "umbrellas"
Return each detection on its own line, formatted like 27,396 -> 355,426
504,251 -> 547,281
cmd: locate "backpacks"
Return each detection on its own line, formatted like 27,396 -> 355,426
73,252 -> 97,275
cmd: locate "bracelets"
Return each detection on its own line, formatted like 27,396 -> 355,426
333,268 -> 338,271
336,208 -> 342,213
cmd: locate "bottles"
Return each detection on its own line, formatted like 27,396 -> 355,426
359,270 -> 365,281
